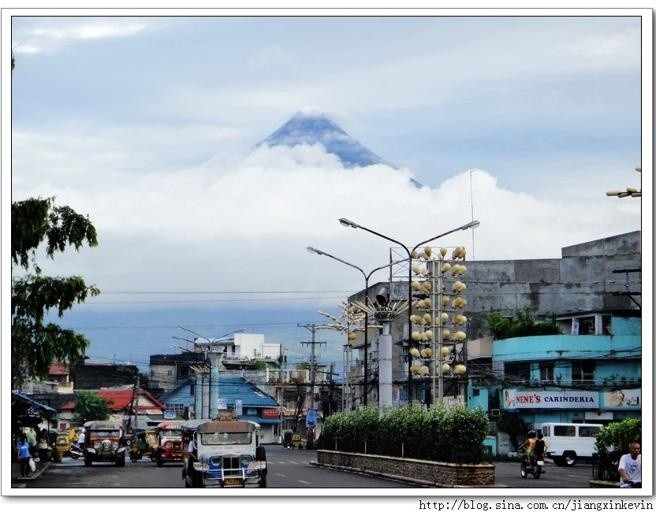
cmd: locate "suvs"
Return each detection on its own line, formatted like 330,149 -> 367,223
82,417 -> 269,488
538,422 -> 616,468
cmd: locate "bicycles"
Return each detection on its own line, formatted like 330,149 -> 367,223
626,481 -> 642,488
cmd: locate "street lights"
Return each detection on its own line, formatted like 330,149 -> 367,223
336,215 -> 486,405
604,166 -> 642,201
403,243 -> 473,406
305,243 -> 428,407
167,323 -> 245,420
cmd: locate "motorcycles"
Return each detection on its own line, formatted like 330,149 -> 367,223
68,442 -> 84,460
519,445 -> 546,481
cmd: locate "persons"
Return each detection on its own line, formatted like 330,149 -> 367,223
618,440 -> 642,488
187,435 -> 197,473
526,433 -> 548,469
519,429 -> 537,468
18,427 -> 85,477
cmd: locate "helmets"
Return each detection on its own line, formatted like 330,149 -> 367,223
528,431 -> 536,438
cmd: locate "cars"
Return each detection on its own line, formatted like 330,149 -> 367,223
40,427 -> 71,456
